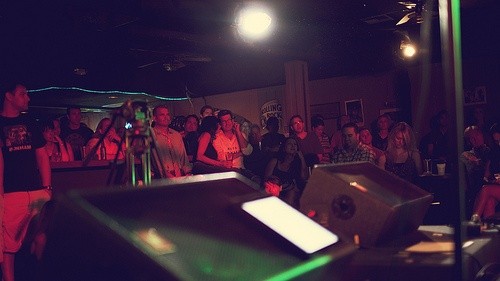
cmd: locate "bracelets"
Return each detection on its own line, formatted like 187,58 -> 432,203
61,144 -> 64,145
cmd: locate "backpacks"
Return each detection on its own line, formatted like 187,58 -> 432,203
183,131 -> 198,156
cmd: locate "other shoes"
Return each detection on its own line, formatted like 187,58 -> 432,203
481,221 -> 495,229
467,214 -> 483,226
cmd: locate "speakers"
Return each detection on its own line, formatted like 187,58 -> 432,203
38,171 -> 362,281
298,160 -> 436,248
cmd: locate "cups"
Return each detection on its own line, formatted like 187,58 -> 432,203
437,163 -> 446,176
424,159 -> 432,174
494,173 -> 500,182
226,154 -> 233,168
51,149 -> 61,162
81,146 -> 90,161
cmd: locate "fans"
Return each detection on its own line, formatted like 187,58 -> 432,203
396,0 -> 438,25
128,48 -> 211,72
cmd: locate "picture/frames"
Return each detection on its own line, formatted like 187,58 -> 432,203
310,102 -> 340,119
463,86 -> 486,105
345,98 -> 364,127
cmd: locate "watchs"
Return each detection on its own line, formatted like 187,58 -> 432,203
44,185 -> 52,190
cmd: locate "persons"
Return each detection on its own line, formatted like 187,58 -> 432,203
0,79 -> 53,281
191,115 -> 232,175
170,104 -> 500,221
36,114 -> 69,162
50,116 -> 75,161
214,110 -> 254,174
61,105 -> 95,161
84,117 -> 127,159
149,106 -> 192,178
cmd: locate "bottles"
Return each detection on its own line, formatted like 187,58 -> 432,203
100,141 -> 107,160
